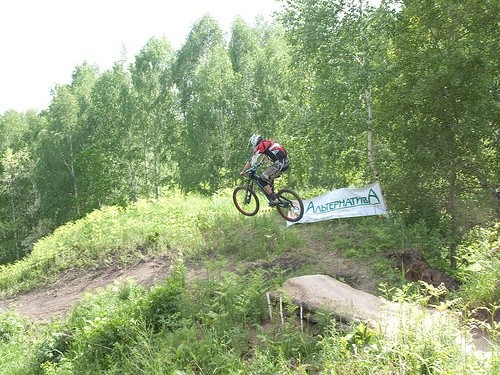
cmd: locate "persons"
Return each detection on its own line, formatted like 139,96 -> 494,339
240,134 -> 290,207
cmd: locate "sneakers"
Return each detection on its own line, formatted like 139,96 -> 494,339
269,198 -> 279,206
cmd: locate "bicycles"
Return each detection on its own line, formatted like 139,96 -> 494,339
233,164 -> 305,222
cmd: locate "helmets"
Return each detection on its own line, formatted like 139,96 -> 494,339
249,135 -> 262,147
